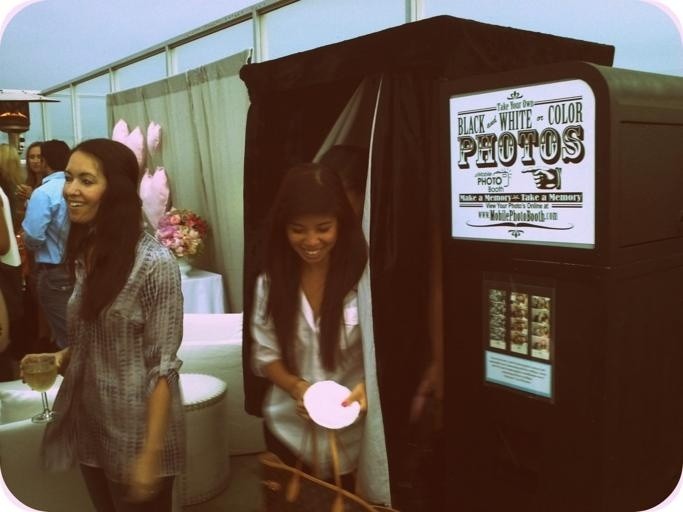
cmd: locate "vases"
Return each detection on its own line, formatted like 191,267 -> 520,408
177,258 -> 193,280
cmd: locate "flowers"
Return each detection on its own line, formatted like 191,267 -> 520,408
155,209 -> 207,258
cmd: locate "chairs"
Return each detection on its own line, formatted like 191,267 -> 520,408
177,310 -> 267,457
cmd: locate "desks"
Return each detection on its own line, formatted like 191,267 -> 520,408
181,268 -> 230,314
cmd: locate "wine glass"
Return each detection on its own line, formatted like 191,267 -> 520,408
21,354 -> 63,423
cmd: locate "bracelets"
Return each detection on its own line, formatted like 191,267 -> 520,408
290,377 -> 304,397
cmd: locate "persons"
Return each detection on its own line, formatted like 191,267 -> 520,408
318,143 -> 369,225
0,138 -> 82,383
247,159 -> 369,511
17,135 -> 191,512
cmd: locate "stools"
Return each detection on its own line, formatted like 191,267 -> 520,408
178,374 -> 231,506
